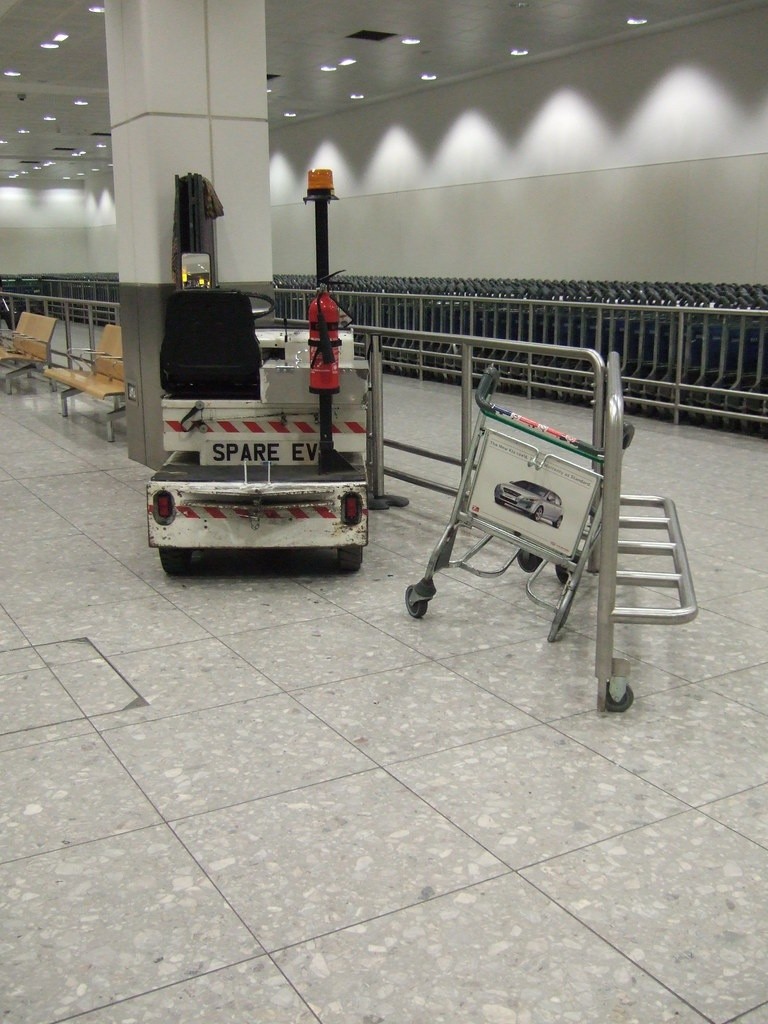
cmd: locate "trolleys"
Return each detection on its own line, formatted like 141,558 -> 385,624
404,365 -> 635,643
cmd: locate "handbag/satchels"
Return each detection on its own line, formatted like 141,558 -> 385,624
161,289 -> 276,399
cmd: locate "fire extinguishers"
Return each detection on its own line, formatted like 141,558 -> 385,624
306,269 -> 354,391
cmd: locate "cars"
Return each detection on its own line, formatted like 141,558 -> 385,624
494,480 -> 564,528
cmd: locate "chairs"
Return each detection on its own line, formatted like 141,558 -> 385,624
160,291 -> 262,399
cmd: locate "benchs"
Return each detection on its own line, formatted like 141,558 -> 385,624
0,312 -> 58,395
44,324 -> 140,442
